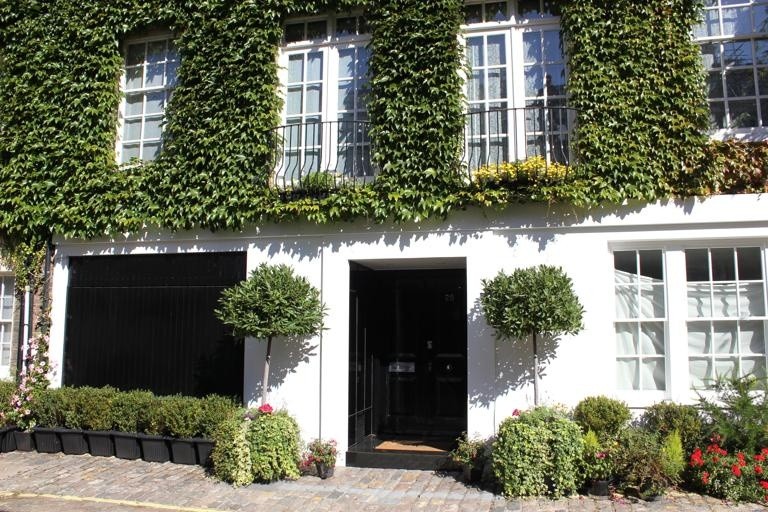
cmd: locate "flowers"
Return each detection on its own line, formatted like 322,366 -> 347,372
447,431 -> 493,470
300,437 -> 338,469
590,453 -> 614,479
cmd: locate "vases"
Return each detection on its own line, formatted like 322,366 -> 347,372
589,478 -> 615,496
315,462 -> 334,479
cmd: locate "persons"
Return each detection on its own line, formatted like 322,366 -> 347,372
533,75 -> 559,145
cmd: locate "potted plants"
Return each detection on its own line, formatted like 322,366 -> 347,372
463,462 -> 482,482
0,376 -> 245,467
